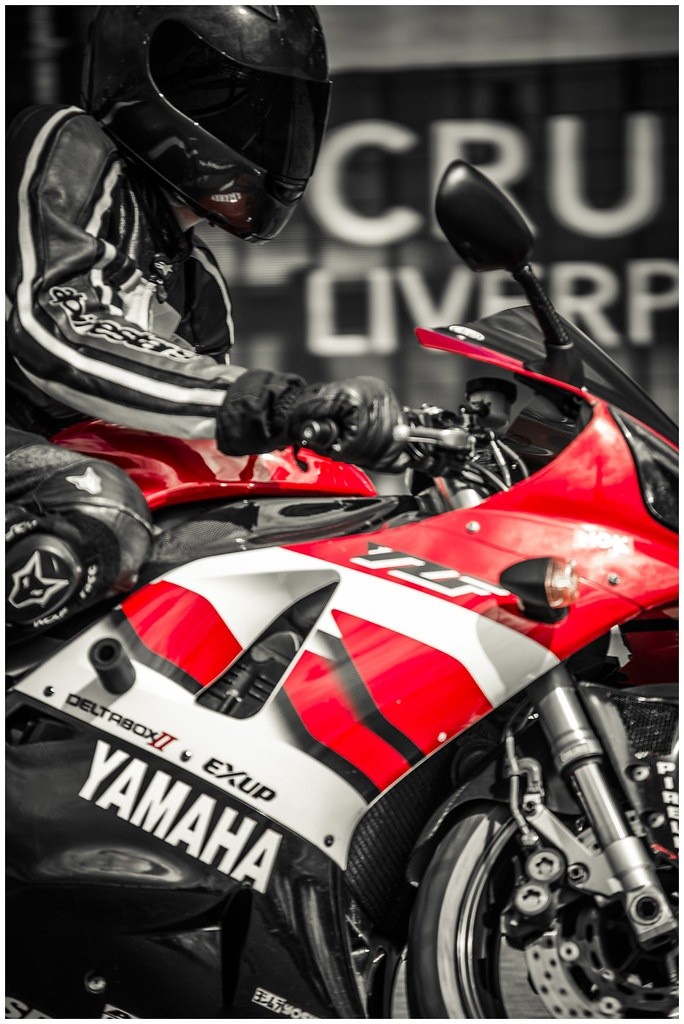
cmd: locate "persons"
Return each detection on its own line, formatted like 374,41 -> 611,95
4,4 -> 408,673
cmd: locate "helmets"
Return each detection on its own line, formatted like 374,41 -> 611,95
80,4 -> 329,246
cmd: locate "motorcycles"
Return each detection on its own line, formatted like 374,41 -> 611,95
7,154 -> 678,1017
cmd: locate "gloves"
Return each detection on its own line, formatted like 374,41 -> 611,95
215,367 -> 410,475
